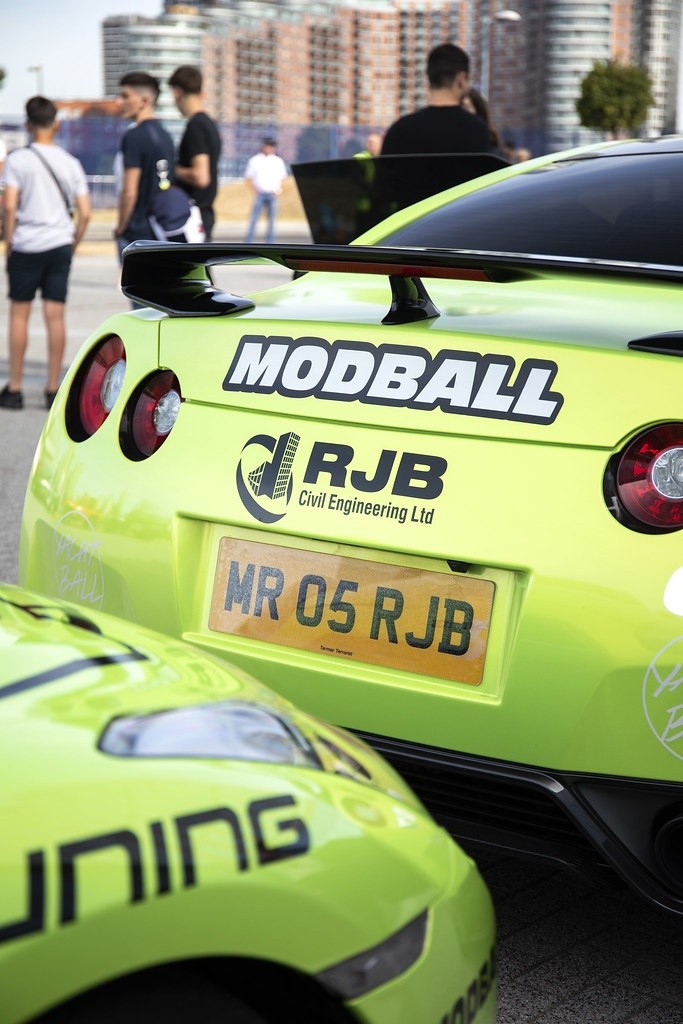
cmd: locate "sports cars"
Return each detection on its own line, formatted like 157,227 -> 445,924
18,135 -> 683,915
0,580 -> 497,1024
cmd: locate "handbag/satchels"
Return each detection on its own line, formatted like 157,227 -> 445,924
147,181 -> 208,246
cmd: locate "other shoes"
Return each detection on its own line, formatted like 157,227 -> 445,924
0,386 -> 23,409
44,389 -> 56,409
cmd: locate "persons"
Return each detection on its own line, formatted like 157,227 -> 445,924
0,96 -> 92,410
343,43 -> 531,227
245,137 -> 290,243
167,64 -> 221,243
112,71 -> 188,311
